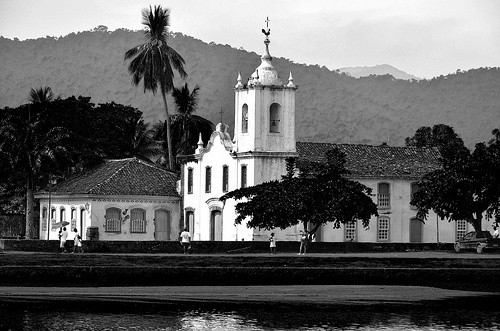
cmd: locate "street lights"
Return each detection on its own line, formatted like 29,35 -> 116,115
47,175 -> 58,240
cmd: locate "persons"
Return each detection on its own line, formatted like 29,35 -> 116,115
179,227 -> 191,254
58,227 -> 68,253
269,233 -> 276,255
71,228 -> 84,253
492,226 -> 499,238
297,231 -> 308,255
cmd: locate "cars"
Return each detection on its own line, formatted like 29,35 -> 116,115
453,230 -> 500,254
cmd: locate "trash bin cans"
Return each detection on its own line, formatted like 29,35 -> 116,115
86,226 -> 99,240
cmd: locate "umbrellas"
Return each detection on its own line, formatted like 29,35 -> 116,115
52,222 -> 69,229
